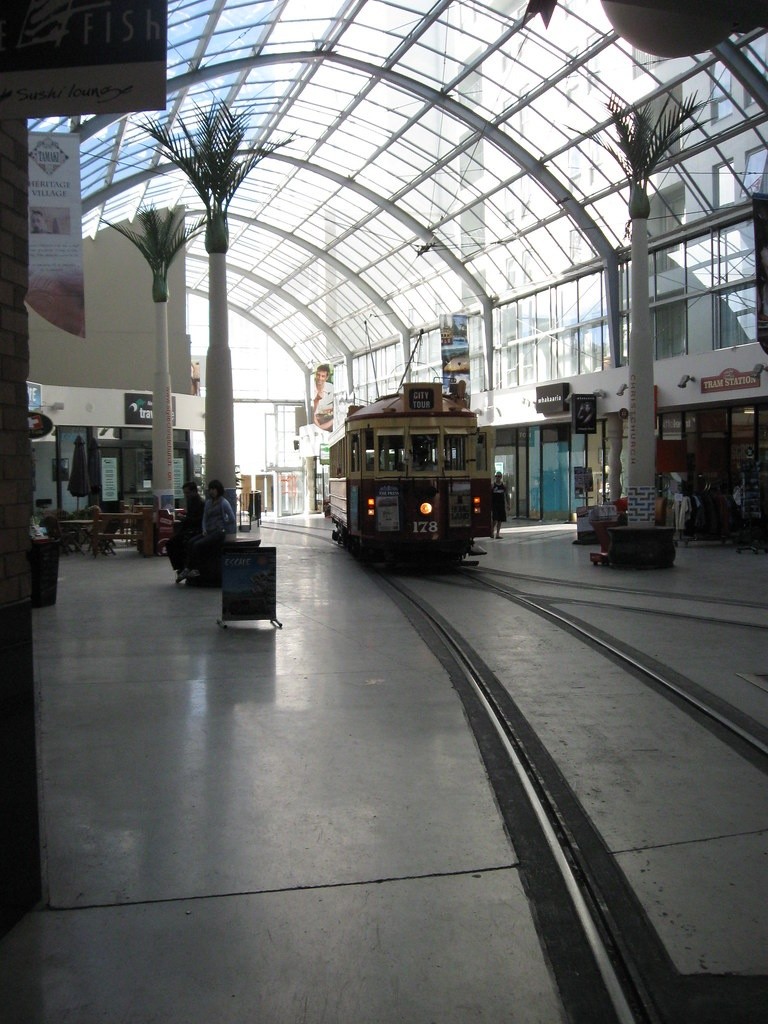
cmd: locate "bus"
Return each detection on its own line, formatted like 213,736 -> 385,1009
331,321 -> 493,575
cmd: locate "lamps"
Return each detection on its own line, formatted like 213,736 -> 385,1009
750,364 -> 768,377
677,375 -> 695,388
616,384 -> 629,396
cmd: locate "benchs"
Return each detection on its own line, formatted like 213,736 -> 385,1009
184,537 -> 262,588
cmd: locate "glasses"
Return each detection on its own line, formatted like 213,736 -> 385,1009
495,475 -> 501,478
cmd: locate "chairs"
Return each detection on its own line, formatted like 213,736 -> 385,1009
44,516 -> 117,557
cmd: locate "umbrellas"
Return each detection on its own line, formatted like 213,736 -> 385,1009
88,437 -> 102,505
67,435 -> 91,512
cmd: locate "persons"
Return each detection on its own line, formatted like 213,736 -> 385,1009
578,402 -> 594,428
311,365 -> 334,414
413,448 -> 433,470
166,482 -> 205,583
178,480 -> 236,577
491,474 -> 511,539
31,211 -> 44,233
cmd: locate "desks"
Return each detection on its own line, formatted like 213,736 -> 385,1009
58,519 -> 102,553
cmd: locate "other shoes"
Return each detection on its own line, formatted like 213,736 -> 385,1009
187,570 -> 200,577
175,568 -> 190,583
496,535 -> 503,539
